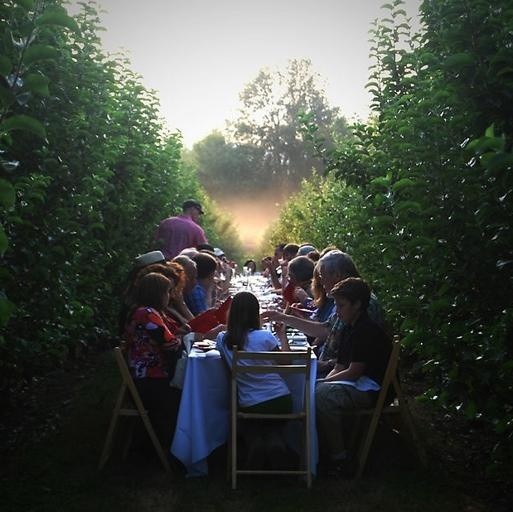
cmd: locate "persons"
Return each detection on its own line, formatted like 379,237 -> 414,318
127,271 -> 182,428
145,199 -> 209,257
213,291 -> 293,474
310,275 -> 403,470
123,238 -> 393,404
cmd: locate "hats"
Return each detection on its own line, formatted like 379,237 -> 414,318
182,199 -> 205,215
214,248 -> 226,257
135,250 -> 166,269
